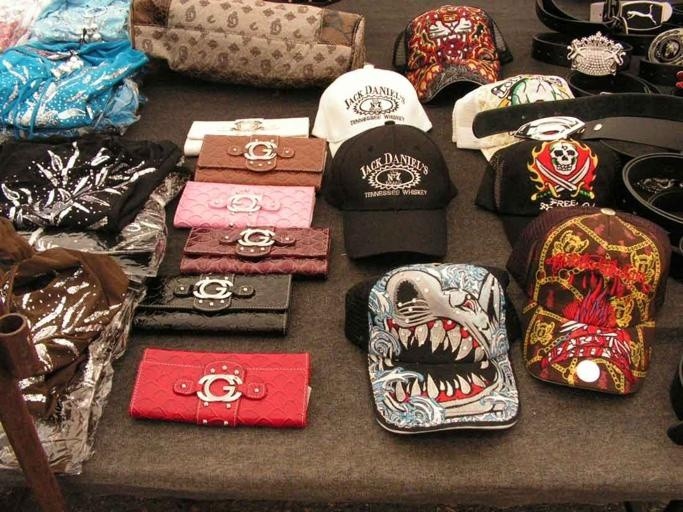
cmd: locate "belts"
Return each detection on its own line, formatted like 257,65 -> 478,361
471,93 -> 681,282
529,0 -> 682,97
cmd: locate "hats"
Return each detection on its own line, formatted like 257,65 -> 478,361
391,5 -> 512,104
344,263 -> 520,435
505,206 -> 671,395
473,138 -> 618,251
450,74 -> 578,162
324,119 -> 457,260
311,64 -> 433,160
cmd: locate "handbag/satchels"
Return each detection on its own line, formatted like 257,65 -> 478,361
128,1 -> 367,91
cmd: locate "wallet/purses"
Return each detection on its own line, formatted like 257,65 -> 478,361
180,227 -> 332,277
128,347 -> 311,427
132,272 -> 291,335
174,181 -> 315,229
183,116 -> 310,157
194,135 -> 328,192
